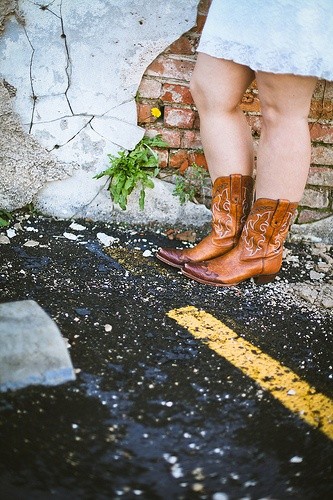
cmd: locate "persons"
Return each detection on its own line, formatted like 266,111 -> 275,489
154,0 -> 333,290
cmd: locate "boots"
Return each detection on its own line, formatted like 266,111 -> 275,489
182,191 -> 299,285
155,175 -> 255,270
